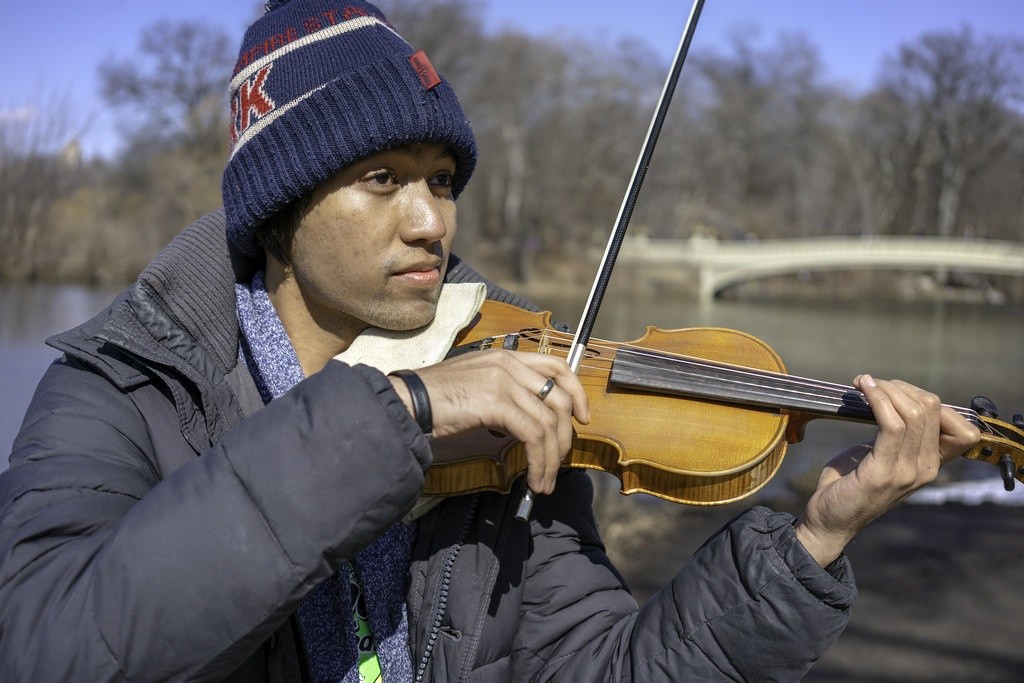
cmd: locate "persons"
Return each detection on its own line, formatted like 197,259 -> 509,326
0,0 -> 983,683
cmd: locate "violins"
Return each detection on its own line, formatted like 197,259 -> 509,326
419,295 -> 1024,506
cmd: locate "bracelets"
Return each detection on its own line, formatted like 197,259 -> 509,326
387,369 -> 433,442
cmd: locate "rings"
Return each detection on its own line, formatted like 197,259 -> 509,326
537,378 -> 555,401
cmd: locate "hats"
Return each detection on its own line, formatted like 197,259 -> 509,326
220,0 -> 480,259
